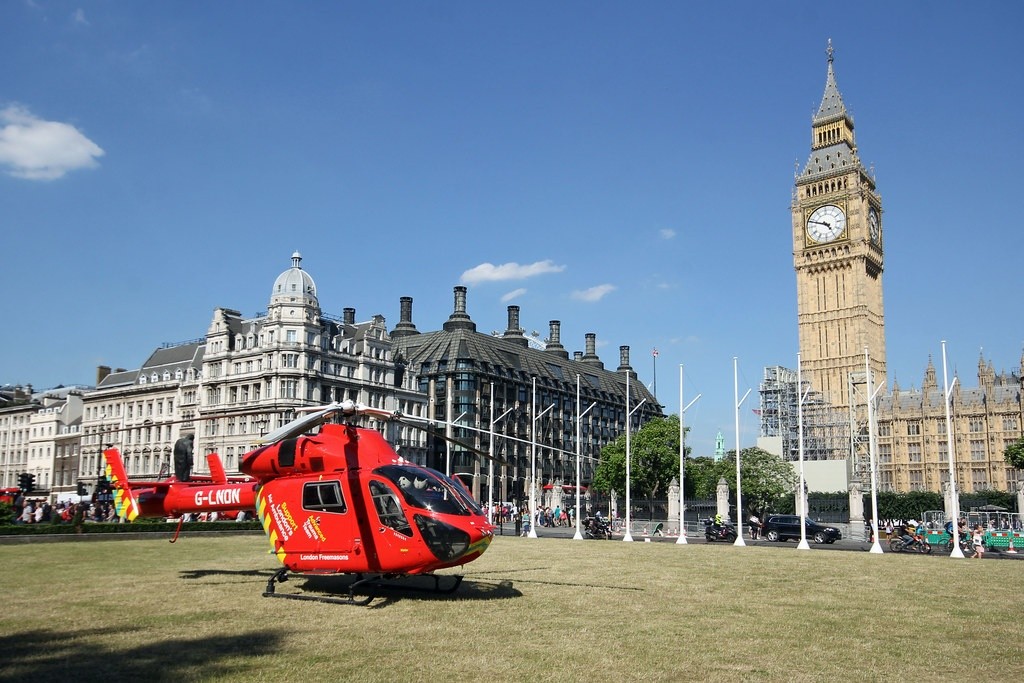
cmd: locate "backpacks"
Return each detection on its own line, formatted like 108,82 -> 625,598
944,521 -> 952,529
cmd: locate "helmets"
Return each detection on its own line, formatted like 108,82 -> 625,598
716,514 -> 721,520
902,520 -> 908,524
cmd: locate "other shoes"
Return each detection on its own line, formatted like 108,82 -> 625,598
752,538 -> 755,540
912,547 -> 917,551
589,532 -> 593,536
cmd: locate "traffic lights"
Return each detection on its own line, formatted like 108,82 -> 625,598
17,472 -> 37,494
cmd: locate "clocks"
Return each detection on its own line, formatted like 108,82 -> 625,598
869,207 -> 879,243
806,204 -> 846,243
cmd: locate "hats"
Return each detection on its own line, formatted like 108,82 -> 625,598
596,511 -> 601,516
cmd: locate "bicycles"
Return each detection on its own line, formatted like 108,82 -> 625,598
937,534 -> 976,555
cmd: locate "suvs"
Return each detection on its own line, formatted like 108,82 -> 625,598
759,514 -> 843,544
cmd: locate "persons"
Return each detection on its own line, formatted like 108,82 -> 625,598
885,515 -> 1020,560
480,501 -> 568,537
748,509 -> 763,540
16,497 -> 119,525
715,513 -> 727,535
612,509 -> 617,521
174,433 -> 195,482
590,511 -> 604,535
387,474 -> 430,535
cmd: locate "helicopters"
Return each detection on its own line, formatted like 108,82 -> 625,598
95,399 -> 612,609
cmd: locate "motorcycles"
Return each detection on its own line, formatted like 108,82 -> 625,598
579,518 -> 612,539
889,534 -> 932,554
702,517 -> 738,543
